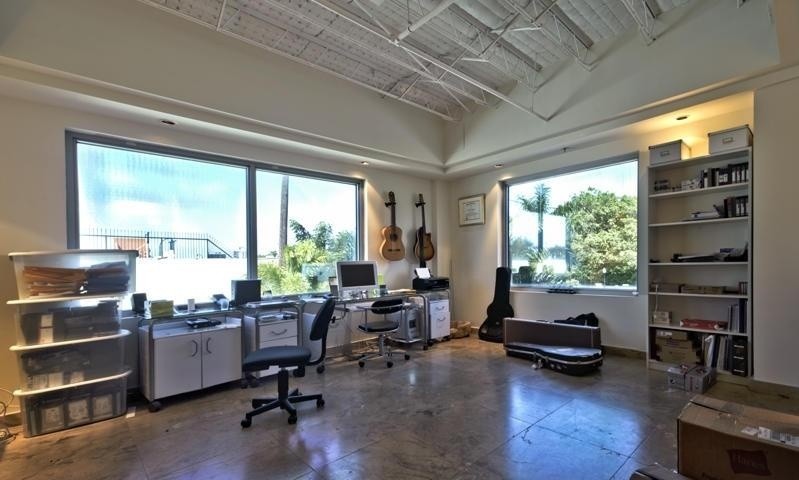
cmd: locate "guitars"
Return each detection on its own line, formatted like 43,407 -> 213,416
379,191 -> 405,261
413,193 -> 434,261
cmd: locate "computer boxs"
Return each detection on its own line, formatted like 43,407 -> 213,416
384,301 -> 420,339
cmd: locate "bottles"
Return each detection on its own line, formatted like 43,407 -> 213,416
379,284 -> 387,297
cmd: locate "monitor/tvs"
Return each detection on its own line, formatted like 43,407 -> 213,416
337,260 -> 379,299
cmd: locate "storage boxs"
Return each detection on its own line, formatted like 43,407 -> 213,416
676,395 -> 799,480
707,124 -> 753,154
5,249 -> 139,438
648,139 -> 692,166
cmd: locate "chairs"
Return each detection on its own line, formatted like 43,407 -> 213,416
241,297 -> 335,428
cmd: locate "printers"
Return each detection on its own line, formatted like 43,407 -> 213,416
412,267 -> 450,291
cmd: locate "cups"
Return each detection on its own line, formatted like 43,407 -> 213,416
143,300 -> 152,320
220,298 -> 230,311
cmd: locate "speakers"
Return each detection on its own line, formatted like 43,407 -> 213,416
330,284 -> 338,298
380,284 -> 388,297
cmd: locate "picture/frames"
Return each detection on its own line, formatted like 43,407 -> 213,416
458,193 -> 486,227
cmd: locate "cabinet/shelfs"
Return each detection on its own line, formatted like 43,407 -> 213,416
645,146 -> 753,387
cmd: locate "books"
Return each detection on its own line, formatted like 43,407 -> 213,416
680,164 -> 749,218
143,299 -> 174,319
700,281 -> 747,373
23,259 -> 130,300
